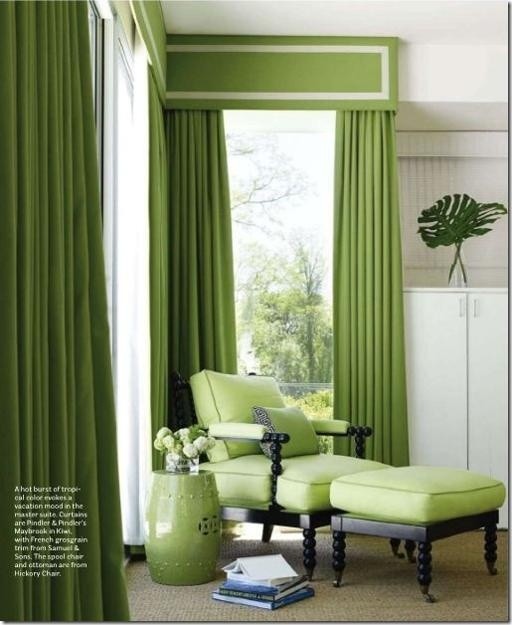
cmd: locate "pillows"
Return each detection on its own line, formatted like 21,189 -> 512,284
253,405 -> 318,457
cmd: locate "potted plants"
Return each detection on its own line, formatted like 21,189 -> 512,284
418,194 -> 506,286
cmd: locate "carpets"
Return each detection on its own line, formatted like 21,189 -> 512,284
124,530 -> 508,621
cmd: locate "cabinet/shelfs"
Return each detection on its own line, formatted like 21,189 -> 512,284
400,289 -> 509,530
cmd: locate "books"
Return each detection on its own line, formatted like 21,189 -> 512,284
210,554 -> 315,611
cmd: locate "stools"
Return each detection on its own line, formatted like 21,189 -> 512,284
330,466 -> 506,604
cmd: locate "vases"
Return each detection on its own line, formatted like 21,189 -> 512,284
166,451 -> 199,474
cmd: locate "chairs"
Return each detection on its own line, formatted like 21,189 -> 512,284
171,370 -> 405,581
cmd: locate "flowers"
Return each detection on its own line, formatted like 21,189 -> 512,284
154,421 -> 215,465
